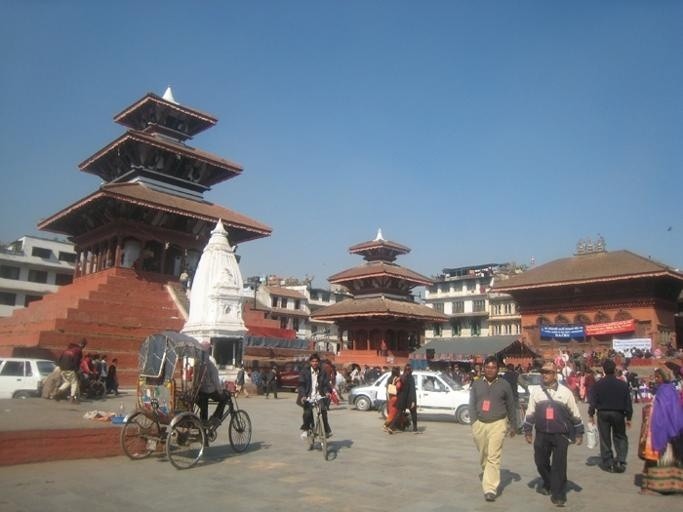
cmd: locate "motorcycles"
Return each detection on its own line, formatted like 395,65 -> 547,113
68,367 -> 107,402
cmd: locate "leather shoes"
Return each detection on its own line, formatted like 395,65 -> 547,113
600,461 -> 625,473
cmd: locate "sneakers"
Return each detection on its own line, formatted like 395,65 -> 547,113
485,493 -> 495,501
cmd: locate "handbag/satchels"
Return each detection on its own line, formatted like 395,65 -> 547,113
565,420 -> 576,444
586,422 -> 600,449
387,384 -> 398,396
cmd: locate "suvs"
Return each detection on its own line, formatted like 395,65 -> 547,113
261,359 -> 337,389
471,369 -> 530,413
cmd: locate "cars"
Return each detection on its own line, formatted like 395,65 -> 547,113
374,370 -> 471,425
517,369 -> 563,408
348,370 -> 404,411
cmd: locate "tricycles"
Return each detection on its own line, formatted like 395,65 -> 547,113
120,329 -> 252,470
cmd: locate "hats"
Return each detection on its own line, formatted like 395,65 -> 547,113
537,361 -> 557,374
200,342 -> 214,351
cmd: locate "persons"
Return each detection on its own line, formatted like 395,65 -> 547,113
195,342 -> 227,445
297,354 -> 336,438
235,365 -> 279,399
555,342 -> 682,473
178,266 -> 188,291
523,359 -> 585,508
467,354 -> 517,501
428,361 -> 533,389
384,365 -> 418,433
335,364 -> 389,400
53,337 -> 119,404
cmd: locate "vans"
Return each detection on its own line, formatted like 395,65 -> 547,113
0,356 -> 63,400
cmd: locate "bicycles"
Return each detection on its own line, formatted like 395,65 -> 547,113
302,396 -> 332,457
